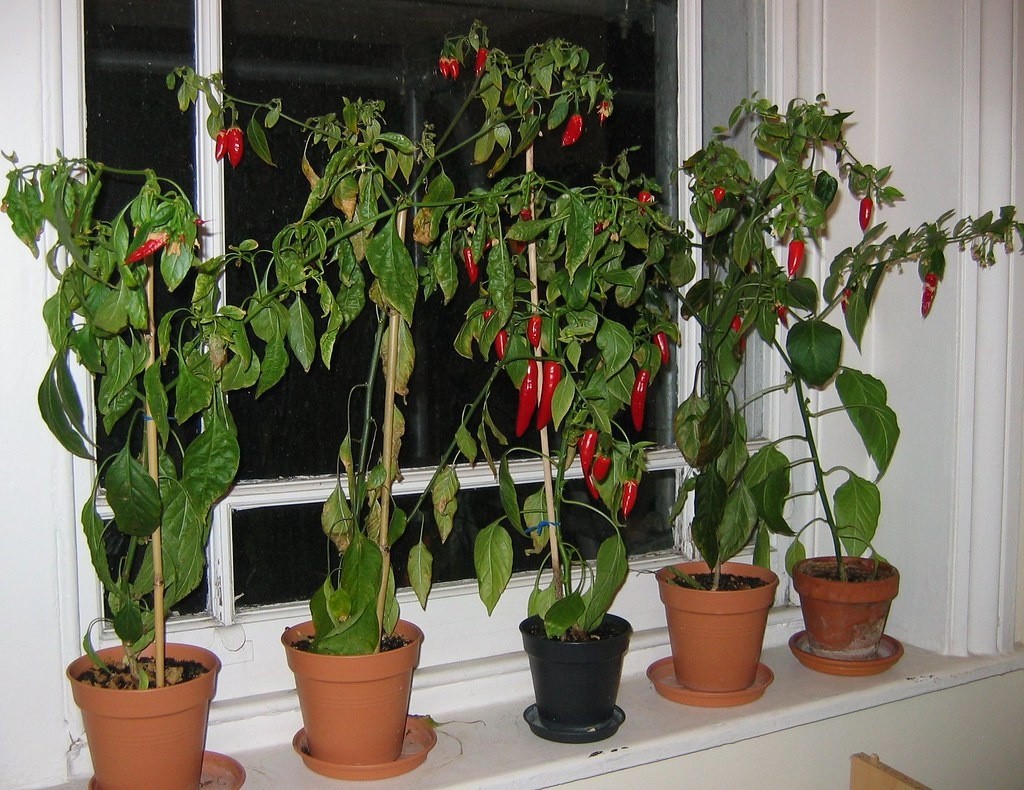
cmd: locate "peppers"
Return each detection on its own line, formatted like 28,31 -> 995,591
123,47 -> 940,522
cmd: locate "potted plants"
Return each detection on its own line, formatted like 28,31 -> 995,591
0,20 -> 1023,790
